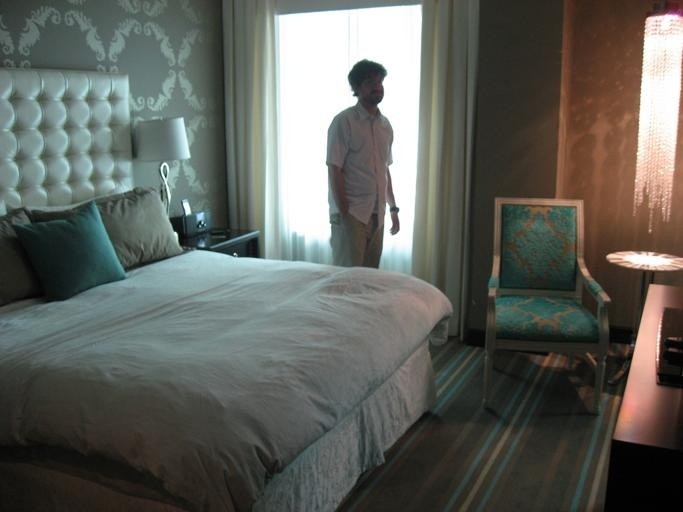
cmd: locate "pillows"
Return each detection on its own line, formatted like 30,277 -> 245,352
24,199 -> 91,225
92,184 -> 184,270
14,201 -> 127,302
0,207 -> 41,307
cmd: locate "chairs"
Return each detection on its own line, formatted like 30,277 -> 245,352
482,197 -> 614,416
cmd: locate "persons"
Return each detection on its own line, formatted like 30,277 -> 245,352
325,59 -> 401,272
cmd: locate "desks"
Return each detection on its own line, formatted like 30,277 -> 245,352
602,250 -> 682,388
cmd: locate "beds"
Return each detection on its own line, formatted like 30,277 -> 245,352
0,66 -> 453,512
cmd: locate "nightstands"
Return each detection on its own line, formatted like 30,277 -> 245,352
181,228 -> 259,258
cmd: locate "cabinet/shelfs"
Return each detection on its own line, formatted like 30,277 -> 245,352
606,284 -> 682,512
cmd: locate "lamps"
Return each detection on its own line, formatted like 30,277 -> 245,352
632,0 -> 682,234
132,116 -> 191,216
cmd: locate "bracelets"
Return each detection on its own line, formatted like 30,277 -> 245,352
389,206 -> 399,214
